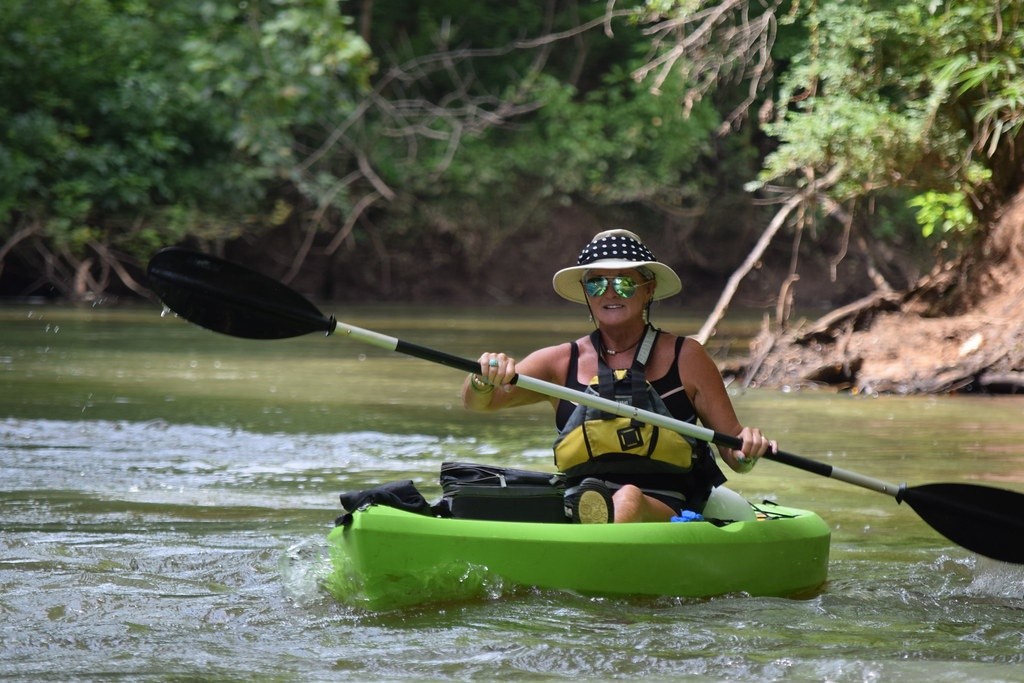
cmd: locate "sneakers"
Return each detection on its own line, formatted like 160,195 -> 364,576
572,476 -> 614,523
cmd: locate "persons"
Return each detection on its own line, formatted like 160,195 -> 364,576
461,229 -> 777,523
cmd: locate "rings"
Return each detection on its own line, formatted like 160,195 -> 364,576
489,359 -> 498,367
761,436 -> 766,439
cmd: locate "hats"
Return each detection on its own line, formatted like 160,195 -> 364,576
553,229 -> 683,305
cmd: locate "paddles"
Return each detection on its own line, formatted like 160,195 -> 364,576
144,243 -> 1024,571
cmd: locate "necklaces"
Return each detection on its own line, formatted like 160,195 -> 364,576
606,341 -> 639,355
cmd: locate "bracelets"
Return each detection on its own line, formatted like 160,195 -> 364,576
744,461 -> 754,465
471,374 -> 493,394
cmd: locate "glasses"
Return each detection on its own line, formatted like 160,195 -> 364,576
584,276 -> 654,299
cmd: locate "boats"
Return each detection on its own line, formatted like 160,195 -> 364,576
326,501 -> 832,613
